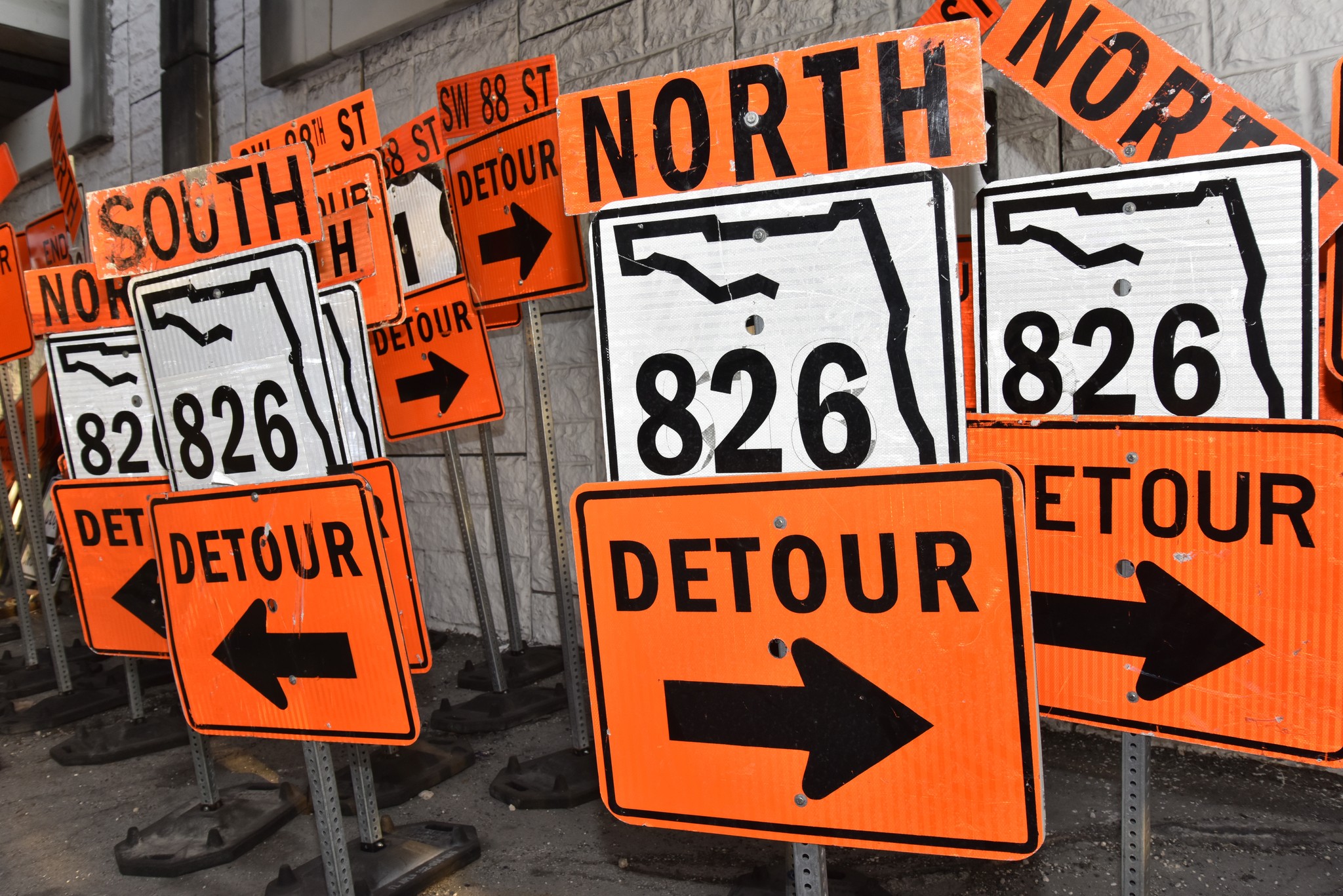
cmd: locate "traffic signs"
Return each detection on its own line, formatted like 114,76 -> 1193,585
444,102 -> 588,311
49,475 -> 171,660
366,272 -> 505,443
568,460 -> 1046,862
145,472 -> 421,747
965,410 -> 1343,774
